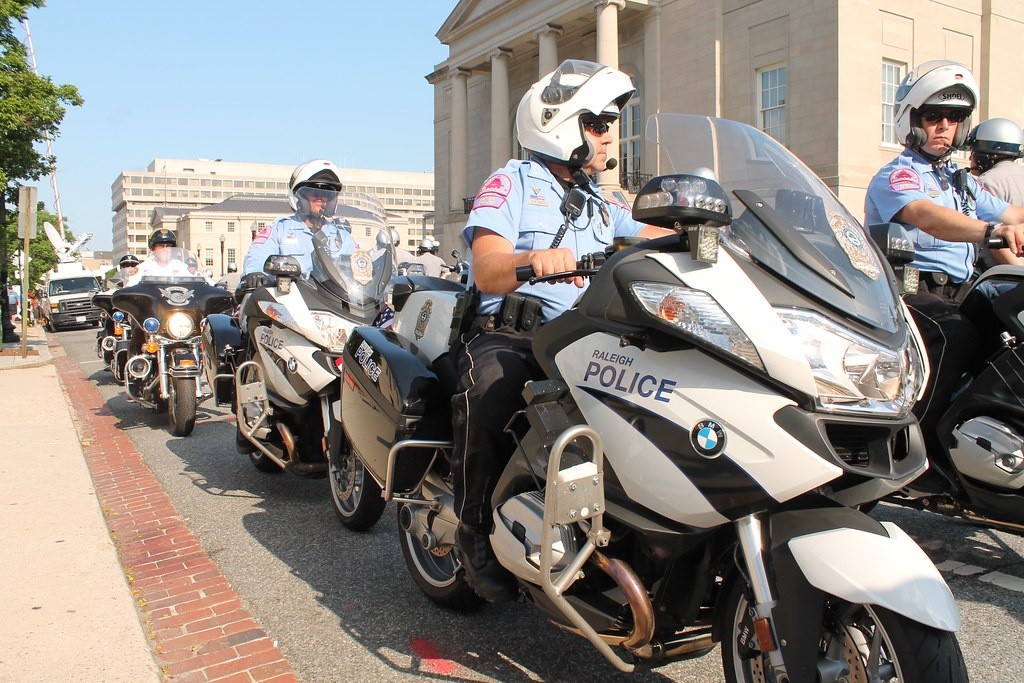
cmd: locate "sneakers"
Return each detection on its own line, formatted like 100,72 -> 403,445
453,522 -> 508,603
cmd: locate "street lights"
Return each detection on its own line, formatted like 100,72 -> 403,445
250,222 -> 257,241
218,234 -> 226,275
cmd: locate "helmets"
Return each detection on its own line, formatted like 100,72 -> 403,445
148,228 -> 177,249
184,257 -> 197,267
205,268 -> 214,277
966,118 -> 1024,158
227,262 -> 238,272
332,217 -> 352,231
893,59 -> 979,149
515,59 -> 637,169
375,228 -> 442,252
119,255 -> 140,266
286,159 -> 344,219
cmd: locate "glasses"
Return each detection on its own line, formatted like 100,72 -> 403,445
121,262 -> 137,267
582,119 -> 612,137
922,109 -> 964,124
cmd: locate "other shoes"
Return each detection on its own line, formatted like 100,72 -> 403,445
234,423 -> 258,455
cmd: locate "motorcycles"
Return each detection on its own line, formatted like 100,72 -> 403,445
440,245 -> 472,282
338,110 -> 969,683
751,117 -> 1024,537
90,246 -> 244,437
198,186 -> 396,532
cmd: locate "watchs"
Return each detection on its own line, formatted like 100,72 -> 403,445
984,220 -> 1004,247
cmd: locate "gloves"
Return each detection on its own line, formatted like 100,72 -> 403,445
245,272 -> 271,289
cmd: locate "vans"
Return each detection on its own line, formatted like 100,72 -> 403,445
40,263 -> 104,333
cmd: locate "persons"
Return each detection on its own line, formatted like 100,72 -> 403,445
185,257 -> 242,300
235,159 -> 365,454
6,286 -> 52,328
864,60 -> 1024,495
332,217 -> 453,279
127,228 -> 228,398
96,255 -> 139,364
449,59 -> 679,600
